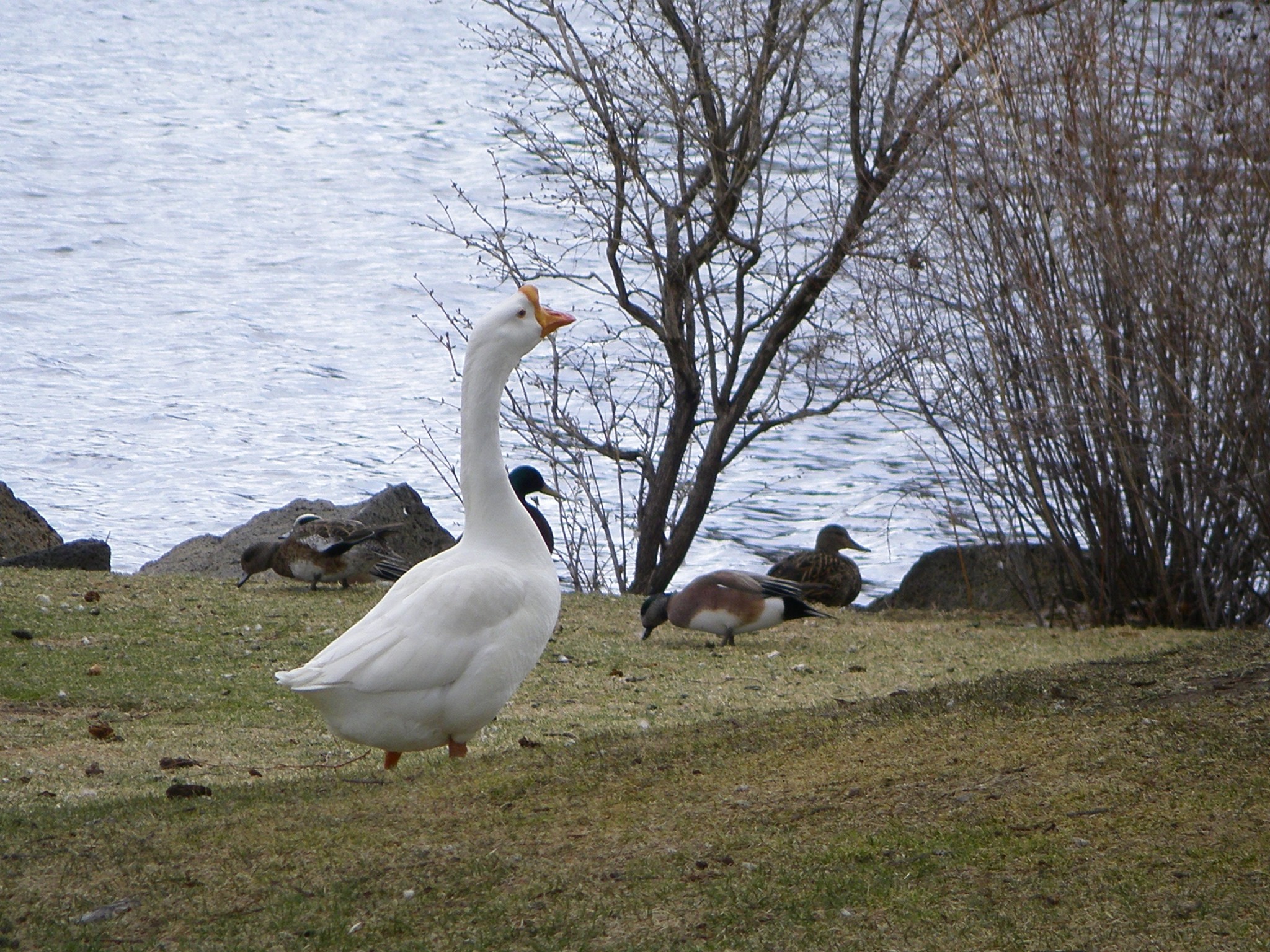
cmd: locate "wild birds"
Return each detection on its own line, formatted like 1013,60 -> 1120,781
640,525 -> 873,648
235,464 -> 565,591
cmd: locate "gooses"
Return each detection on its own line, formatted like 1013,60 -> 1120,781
274,285 -> 561,770
